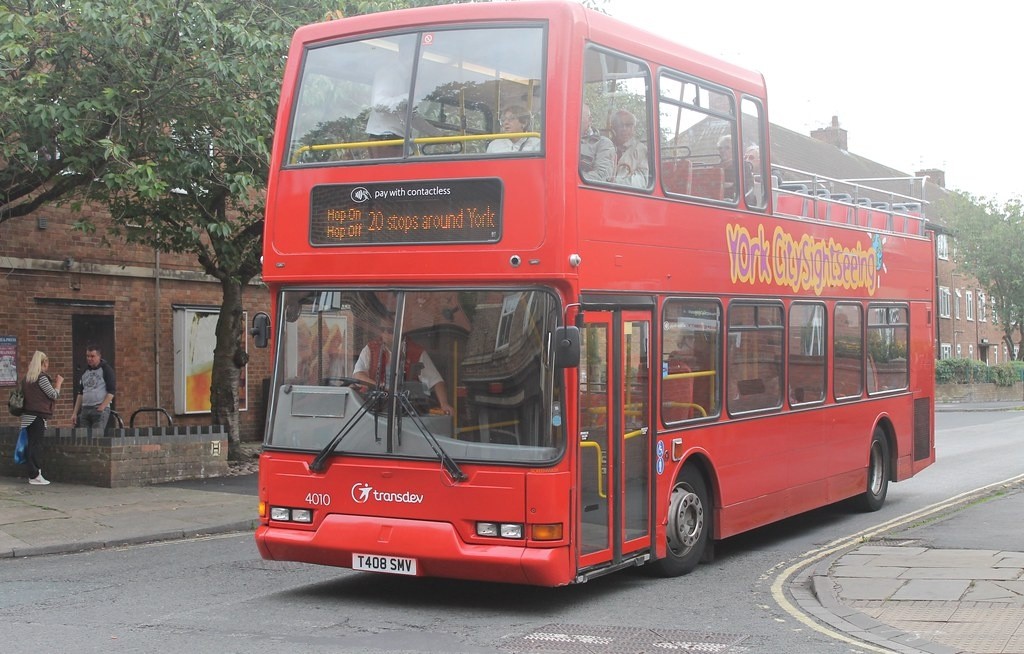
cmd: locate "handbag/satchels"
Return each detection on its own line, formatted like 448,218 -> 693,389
14,429 -> 29,462
8,390 -> 24,417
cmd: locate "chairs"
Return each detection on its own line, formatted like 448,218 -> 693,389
582,361 -> 822,425
660,148 -> 924,237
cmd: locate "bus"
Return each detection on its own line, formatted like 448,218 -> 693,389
249,0 -> 938,587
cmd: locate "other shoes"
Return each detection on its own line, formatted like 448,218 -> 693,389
29,469 -> 50,485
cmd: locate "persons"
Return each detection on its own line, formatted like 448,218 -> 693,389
19,351 -> 64,485
352,311 -> 454,417
364,37 -> 447,159
70,345 -> 116,437
835,314 -> 848,327
609,110 -> 649,188
485,103 -> 541,154
578,104 -> 616,182
716,135 -> 761,206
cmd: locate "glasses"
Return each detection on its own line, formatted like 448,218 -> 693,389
379,327 -> 394,334
744,155 -> 760,160
501,116 -> 518,121
